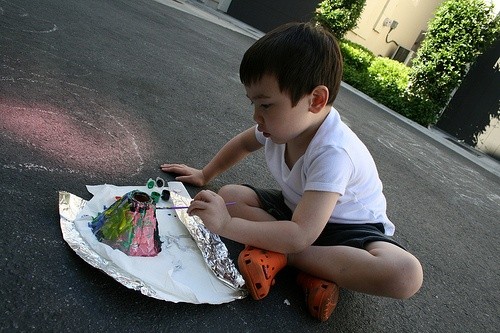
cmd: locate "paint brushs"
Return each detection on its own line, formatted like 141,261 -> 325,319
156,201 -> 237,210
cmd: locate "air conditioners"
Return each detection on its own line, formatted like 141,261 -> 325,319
391,46 -> 416,67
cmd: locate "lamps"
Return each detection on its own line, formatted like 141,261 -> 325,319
386,21 -> 398,46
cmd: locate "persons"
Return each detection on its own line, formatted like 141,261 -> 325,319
160,22 -> 424,321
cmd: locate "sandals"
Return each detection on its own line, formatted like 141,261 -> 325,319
239,245 -> 287,301
299,272 -> 338,321
